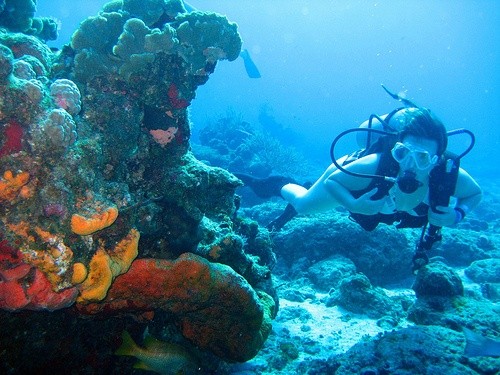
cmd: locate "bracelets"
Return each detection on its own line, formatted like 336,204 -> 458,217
454,207 -> 465,224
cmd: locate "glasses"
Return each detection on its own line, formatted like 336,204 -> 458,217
391,142 -> 437,170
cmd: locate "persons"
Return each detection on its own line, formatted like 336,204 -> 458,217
230,111 -> 485,229
